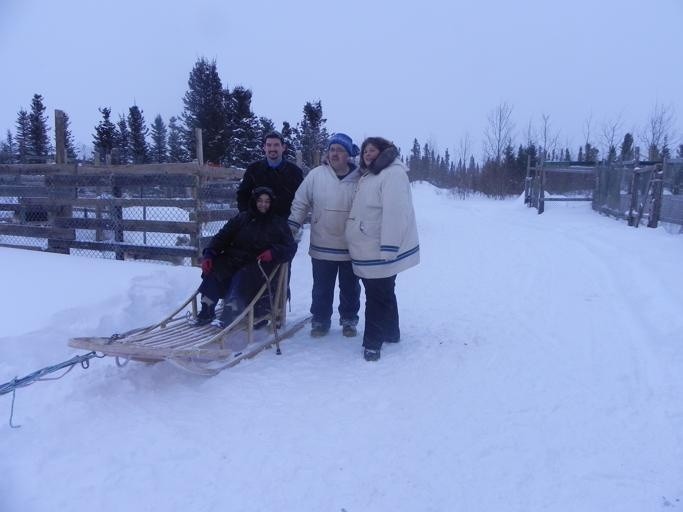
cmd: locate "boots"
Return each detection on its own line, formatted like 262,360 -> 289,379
217,306 -> 233,328
191,302 -> 216,326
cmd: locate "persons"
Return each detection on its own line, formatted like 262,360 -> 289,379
288,133 -> 366,337
187,186 -> 296,331
343,137 -> 420,362
235,132 -> 303,328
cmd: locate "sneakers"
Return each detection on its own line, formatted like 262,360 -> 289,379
365,348 -> 380,361
311,326 -> 328,337
343,326 -> 357,337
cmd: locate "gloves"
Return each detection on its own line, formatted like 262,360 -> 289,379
257,250 -> 272,262
201,259 -> 212,275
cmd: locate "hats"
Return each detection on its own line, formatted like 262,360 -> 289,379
329,133 -> 360,156
